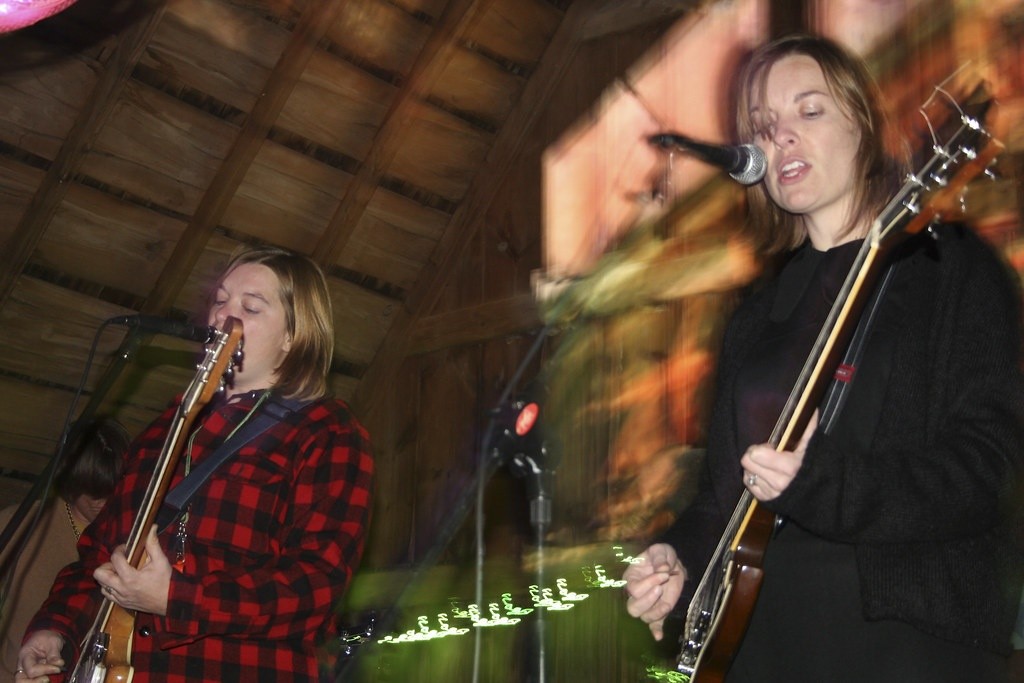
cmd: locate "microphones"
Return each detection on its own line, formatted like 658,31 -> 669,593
111,314 -> 219,344
645,131 -> 768,186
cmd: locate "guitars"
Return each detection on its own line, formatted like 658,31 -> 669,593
643,75 -> 1006,682
60,315 -> 247,683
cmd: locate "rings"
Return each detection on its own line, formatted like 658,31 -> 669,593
748,474 -> 758,485
106,586 -> 111,594
14,670 -> 24,676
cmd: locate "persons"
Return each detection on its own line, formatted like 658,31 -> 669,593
12,244 -> 376,683
621,36 -> 1024,683
